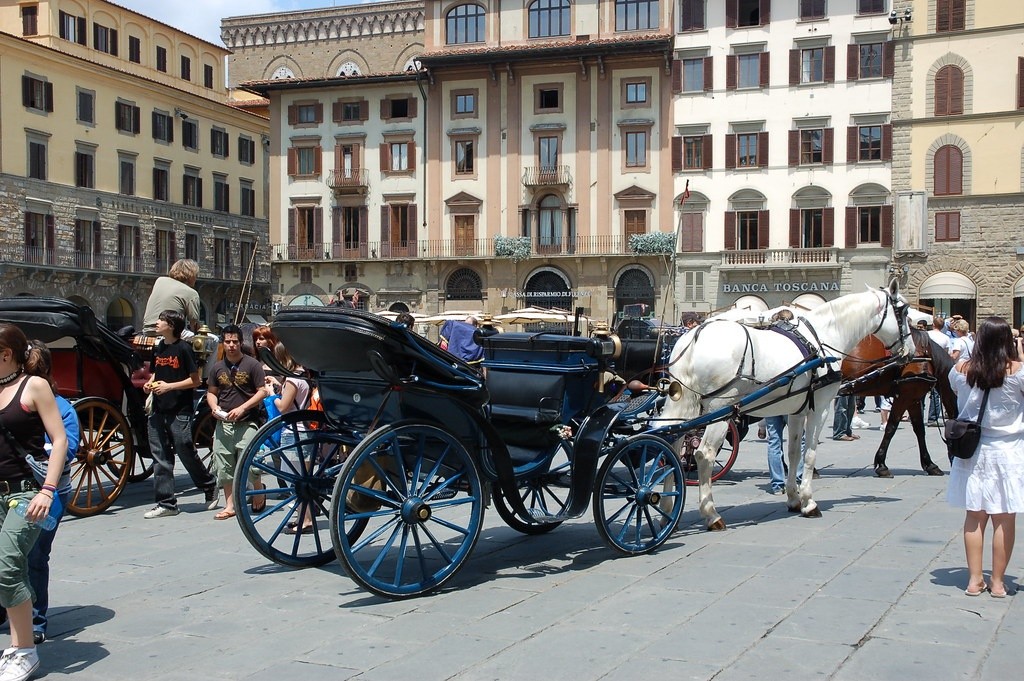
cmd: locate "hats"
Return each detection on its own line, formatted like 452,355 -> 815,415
680,312 -> 705,322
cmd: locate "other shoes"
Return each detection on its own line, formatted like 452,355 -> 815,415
282,522 -> 311,533
33,615 -> 48,642
929,420 -> 945,427
880,422 -> 888,430
851,418 -> 870,429
837,432 -> 859,441
758,426 -> 766,440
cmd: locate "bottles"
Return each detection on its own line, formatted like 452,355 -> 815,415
8,499 -> 58,531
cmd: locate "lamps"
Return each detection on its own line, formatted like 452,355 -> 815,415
501,290 -> 507,298
889,262 -> 909,280
888,8 -> 913,38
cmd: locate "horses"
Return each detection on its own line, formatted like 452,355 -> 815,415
654,277 -> 917,532
679,313 -> 958,478
234,290 -> 358,366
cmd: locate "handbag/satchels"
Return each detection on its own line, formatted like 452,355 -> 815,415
946,418 -> 980,458
26,455 -> 72,495
262,396 -> 282,448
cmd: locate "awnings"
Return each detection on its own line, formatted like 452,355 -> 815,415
921,272 -> 977,299
1013,277 -> 1024,298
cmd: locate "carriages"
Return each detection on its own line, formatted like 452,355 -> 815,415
1,260 -> 273,518
231,273 -> 919,600
611,325 -> 961,480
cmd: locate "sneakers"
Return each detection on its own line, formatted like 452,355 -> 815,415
144,505 -> 179,518
0,645 -> 41,681
205,476 -> 220,510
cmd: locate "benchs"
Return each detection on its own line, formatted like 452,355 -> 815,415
486,370 -> 566,424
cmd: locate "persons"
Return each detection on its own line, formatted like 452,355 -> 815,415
833,390 -> 912,442
241,323 -> 326,535
0,322 -> 68,681
465,316 -> 477,328
389,302 -> 409,313
757,418 -> 767,439
948,316 -> 1023,597
142,309 -> 220,520
916,313 -> 1024,427
0,341 -> 79,643
764,415 -> 807,496
397,314 -> 416,331
143,258 -> 220,389
208,323 -> 269,520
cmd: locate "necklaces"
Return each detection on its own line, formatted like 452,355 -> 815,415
0,369 -> 21,385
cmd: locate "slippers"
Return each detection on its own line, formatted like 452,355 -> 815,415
214,509 -> 235,520
964,580 -> 987,596
251,483 -> 266,512
990,583 -> 1008,597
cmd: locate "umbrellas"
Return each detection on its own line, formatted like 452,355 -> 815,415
375,304 -> 595,341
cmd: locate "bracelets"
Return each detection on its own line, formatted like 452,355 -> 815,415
42,484 -> 56,491
44,482 -> 56,488
39,491 -> 53,499
